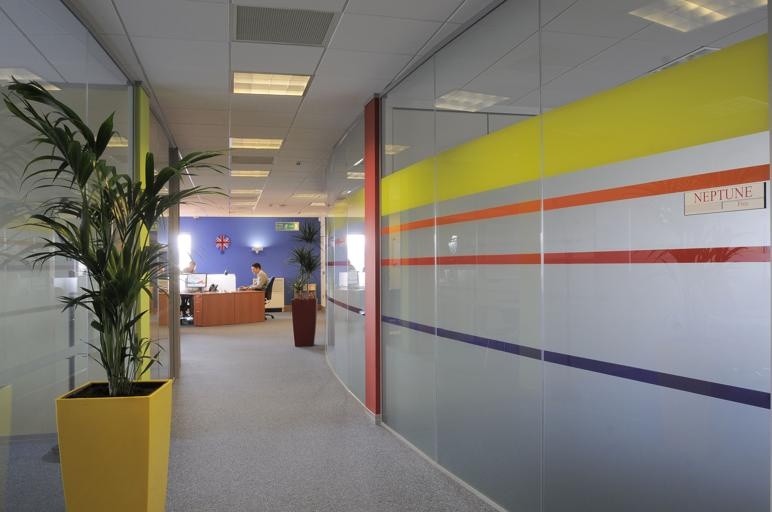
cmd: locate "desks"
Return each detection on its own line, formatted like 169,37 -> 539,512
158,290 -> 265,326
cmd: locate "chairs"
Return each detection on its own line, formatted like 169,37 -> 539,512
255,275 -> 276,321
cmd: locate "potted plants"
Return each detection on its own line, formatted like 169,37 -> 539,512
287,218 -> 325,347
1,73 -> 232,512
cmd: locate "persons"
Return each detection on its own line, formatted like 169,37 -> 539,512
240,262 -> 269,291
181,261 -> 196,274
